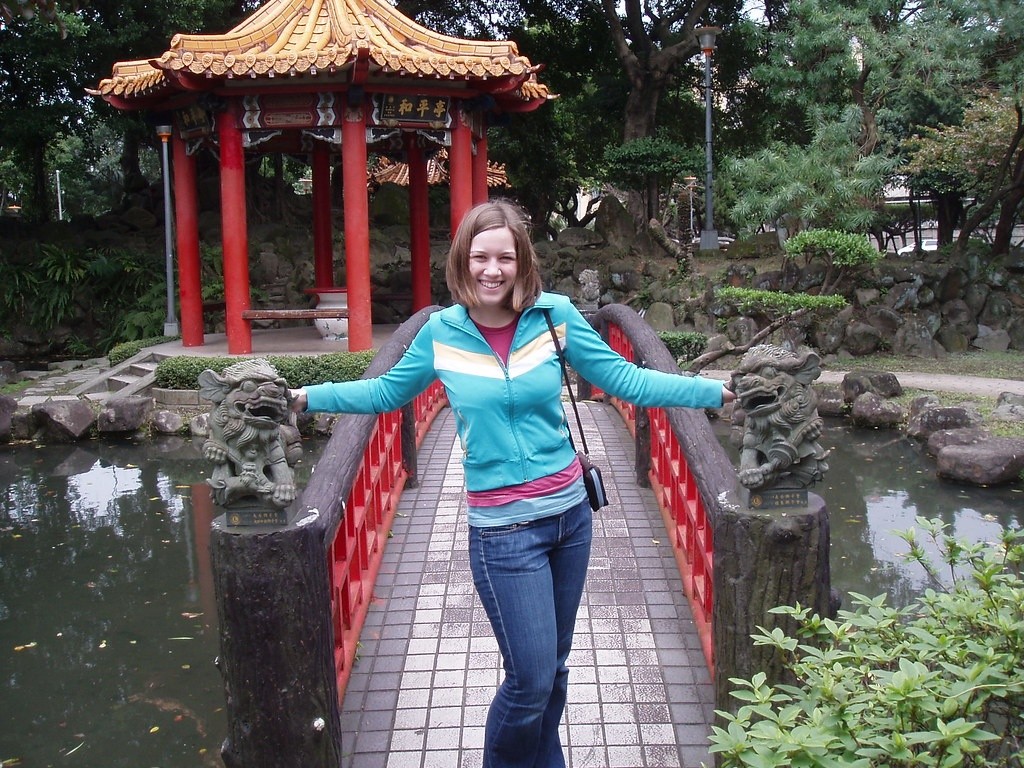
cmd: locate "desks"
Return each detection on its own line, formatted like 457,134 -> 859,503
304,287 -> 348,342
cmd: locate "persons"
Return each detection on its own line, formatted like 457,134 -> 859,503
283,196 -> 738,766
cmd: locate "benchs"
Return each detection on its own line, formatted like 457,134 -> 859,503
371,292 -> 412,301
202,300 -> 226,311
242,309 -> 348,320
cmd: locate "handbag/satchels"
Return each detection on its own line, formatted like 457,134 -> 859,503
577,452 -> 608,513
